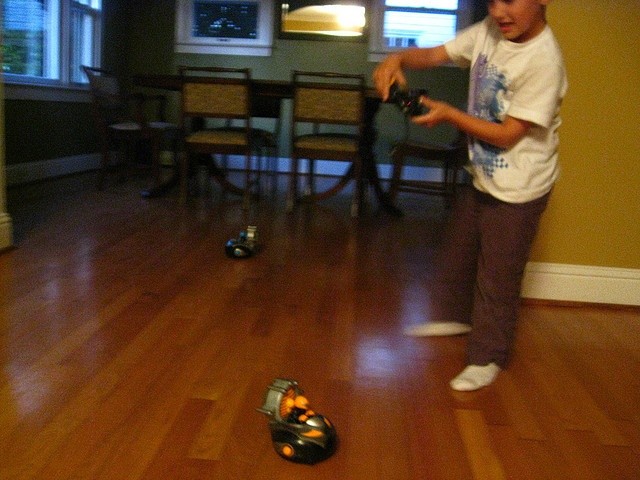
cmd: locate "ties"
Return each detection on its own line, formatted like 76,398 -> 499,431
175,1 -> 276,56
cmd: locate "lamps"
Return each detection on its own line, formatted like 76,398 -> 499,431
382,116 -> 463,208
79,64 -> 180,198
178,66 -> 278,211
284,69 -> 365,219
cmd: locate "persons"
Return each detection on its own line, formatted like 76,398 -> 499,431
371,1 -> 569,393
